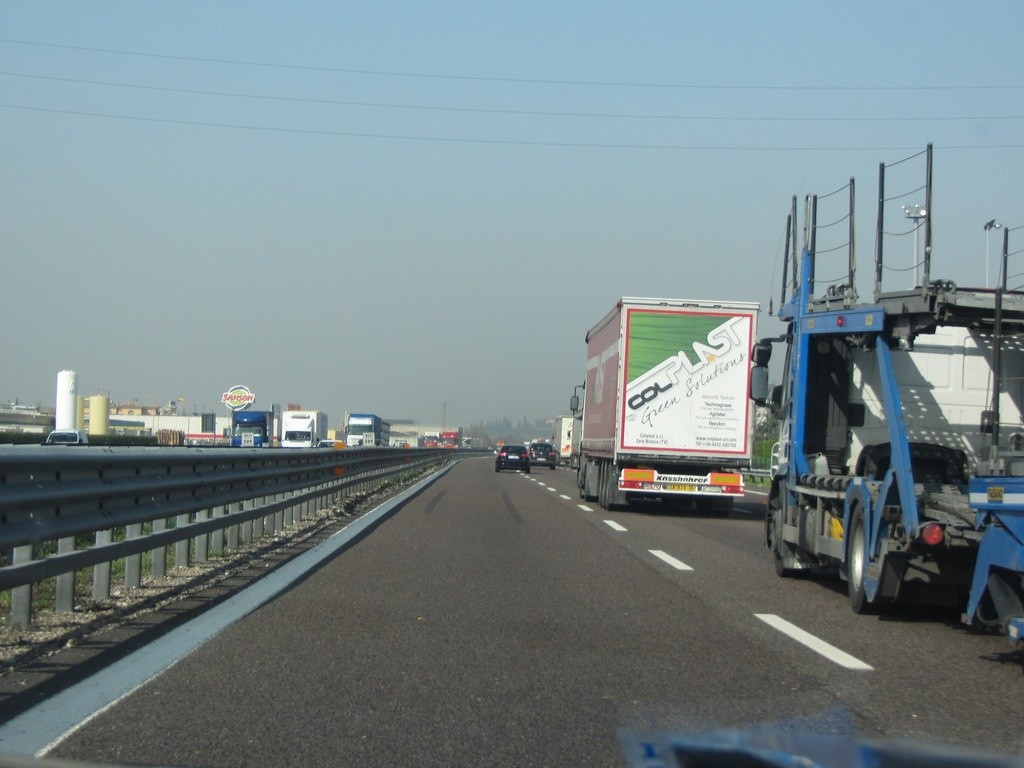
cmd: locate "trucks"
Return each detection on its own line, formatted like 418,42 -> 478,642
442,432 -> 460,449
231,410 -> 274,448
552,407 -> 583,468
749,141 -> 1024,642
346,414 -> 390,447
424,431 -> 442,448
281,411 -> 329,448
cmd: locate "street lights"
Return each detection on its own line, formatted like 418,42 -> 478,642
983,219 -> 1002,288
902,205 -> 925,288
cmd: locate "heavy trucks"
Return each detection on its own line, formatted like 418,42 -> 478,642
570,296 -> 759,518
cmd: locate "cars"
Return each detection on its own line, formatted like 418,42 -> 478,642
494,446 -> 530,473
46,430 -> 88,444
528,442 -> 556,470
313,440 -> 342,448
